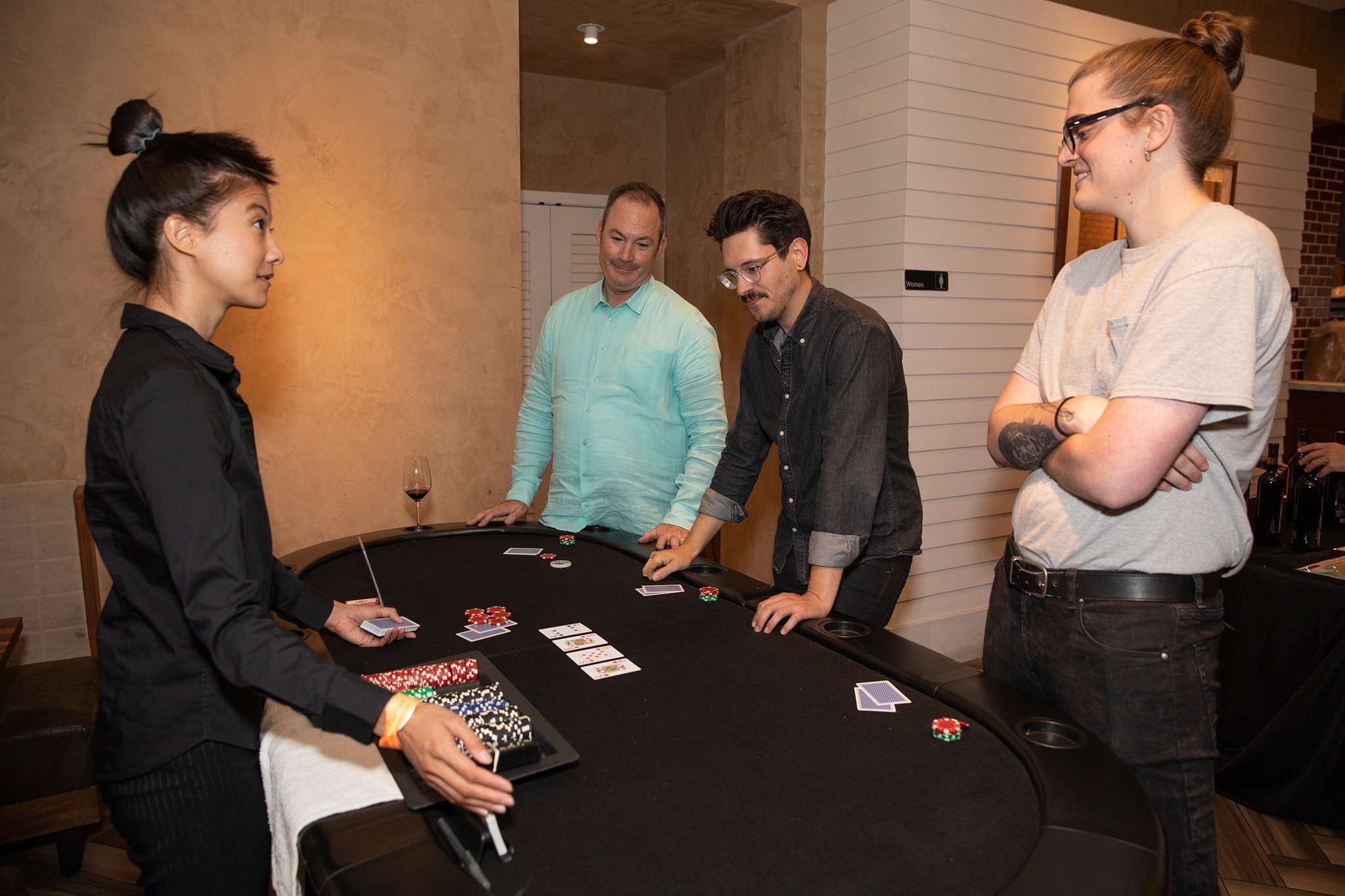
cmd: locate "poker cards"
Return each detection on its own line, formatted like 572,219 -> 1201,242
854,681 -> 912,713
634,584 -> 685,597
456,619 -> 518,643
538,622 -> 642,681
360,616 -> 421,637
503,547 -> 544,556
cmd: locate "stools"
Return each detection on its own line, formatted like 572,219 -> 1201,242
0,657 -> 99,877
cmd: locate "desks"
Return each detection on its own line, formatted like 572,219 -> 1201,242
260,520 -> 1173,896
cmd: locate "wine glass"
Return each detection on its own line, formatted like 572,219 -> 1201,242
402,456 -> 432,530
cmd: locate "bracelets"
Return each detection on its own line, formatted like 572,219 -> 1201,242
378,691 -> 419,750
1054,396 -> 1078,438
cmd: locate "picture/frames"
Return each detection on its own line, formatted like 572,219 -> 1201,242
1053,156 -> 1239,280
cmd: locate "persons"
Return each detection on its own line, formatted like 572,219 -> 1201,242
1296,442 -> 1345,477
639,188 -> 927,634
973,8 -> 1293,895
67,95 -> 517,895
462,180 -> 729,550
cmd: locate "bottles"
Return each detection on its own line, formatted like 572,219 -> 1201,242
1253,427 -> 1345,552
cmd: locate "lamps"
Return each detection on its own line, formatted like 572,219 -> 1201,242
583,25 -> 599,45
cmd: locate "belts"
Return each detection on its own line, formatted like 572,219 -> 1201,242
1003,532 -> 1222,603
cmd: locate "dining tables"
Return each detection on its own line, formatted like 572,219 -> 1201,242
1213,497 -> 1345,830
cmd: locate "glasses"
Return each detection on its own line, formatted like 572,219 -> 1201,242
1061,100 -> 1155,155
716,244 -> 791,290
